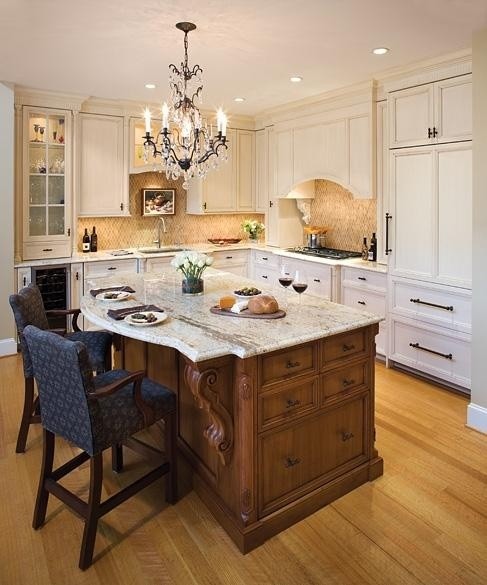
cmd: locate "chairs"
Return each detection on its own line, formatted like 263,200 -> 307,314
21,322 -> 181,572
8,281 -> 119,457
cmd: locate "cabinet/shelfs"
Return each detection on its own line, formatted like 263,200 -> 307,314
215,249 -> 247,277
384,71 -> 471,152
21,104 -> 74,261
385,146 -> 472,393
339,265 -> 385,357
247,249 -> 336,306
78,111 -> 131,221
256,130 -> 280,212
182,124 -> 255,216
254,327 -> 367,517
81,259 -> 139,275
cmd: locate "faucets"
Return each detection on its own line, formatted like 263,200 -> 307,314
154,218 -> 168,249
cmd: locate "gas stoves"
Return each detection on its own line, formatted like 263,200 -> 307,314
286,247 -> 362,259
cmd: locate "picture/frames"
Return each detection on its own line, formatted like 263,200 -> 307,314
139,186 -> 176,217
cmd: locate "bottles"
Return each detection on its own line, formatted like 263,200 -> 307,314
81,225 -> 97,252
367,232 -> 376,260
361,237 -> 368,260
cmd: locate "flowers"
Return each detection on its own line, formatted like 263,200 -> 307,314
170,245 -> 215,276
241,218 -> 267,234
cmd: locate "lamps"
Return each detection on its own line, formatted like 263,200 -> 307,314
135,21 -> 233,181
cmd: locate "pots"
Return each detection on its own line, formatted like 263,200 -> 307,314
303,227 -> 327,248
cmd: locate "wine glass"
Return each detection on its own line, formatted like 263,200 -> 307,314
278,263 -> 293,308
293,269 -> 306,310
31,124 -> 45,142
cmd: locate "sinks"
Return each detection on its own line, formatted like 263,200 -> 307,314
138,249 -> 194,254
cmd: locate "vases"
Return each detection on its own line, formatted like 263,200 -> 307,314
249,235 -> 260,246
181,278 -> 204,294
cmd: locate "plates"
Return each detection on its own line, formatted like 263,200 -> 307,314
127,312 -> 167,325
96,291 -> 131,301
234,288 -> 261,297
